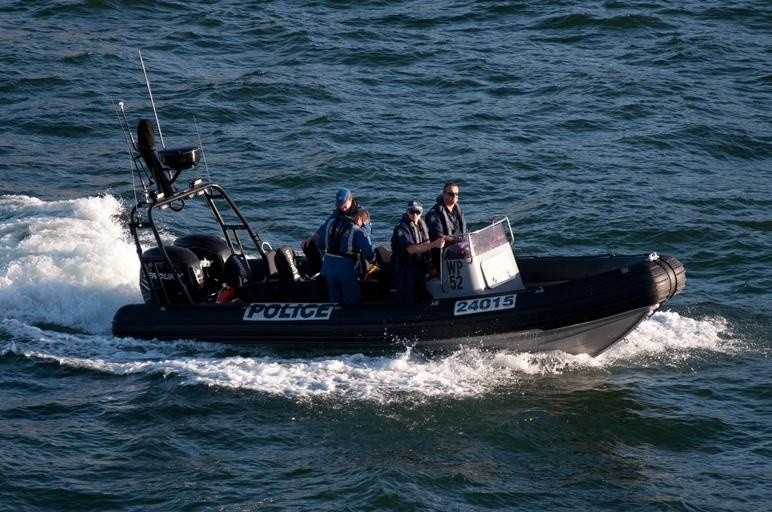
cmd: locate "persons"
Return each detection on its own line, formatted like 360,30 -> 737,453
426,182 -> 466,277
391,199 -> 445,305
322,208 -> 377,304
302,190 -> 372,279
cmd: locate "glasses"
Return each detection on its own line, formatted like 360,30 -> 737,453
444,191 -> 459,196
408,211 -> 421,215
361,218 -> 366,228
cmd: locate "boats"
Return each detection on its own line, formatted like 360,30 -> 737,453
111,232 -> 685,358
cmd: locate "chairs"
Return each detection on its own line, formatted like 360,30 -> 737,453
374,245 -> 391,266
302,239 -> 322,270
274,246 -> 321,287
262,250 -> 276,282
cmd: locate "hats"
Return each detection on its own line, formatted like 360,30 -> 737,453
336,187 -> 351,207
407,199 -> 424,214
